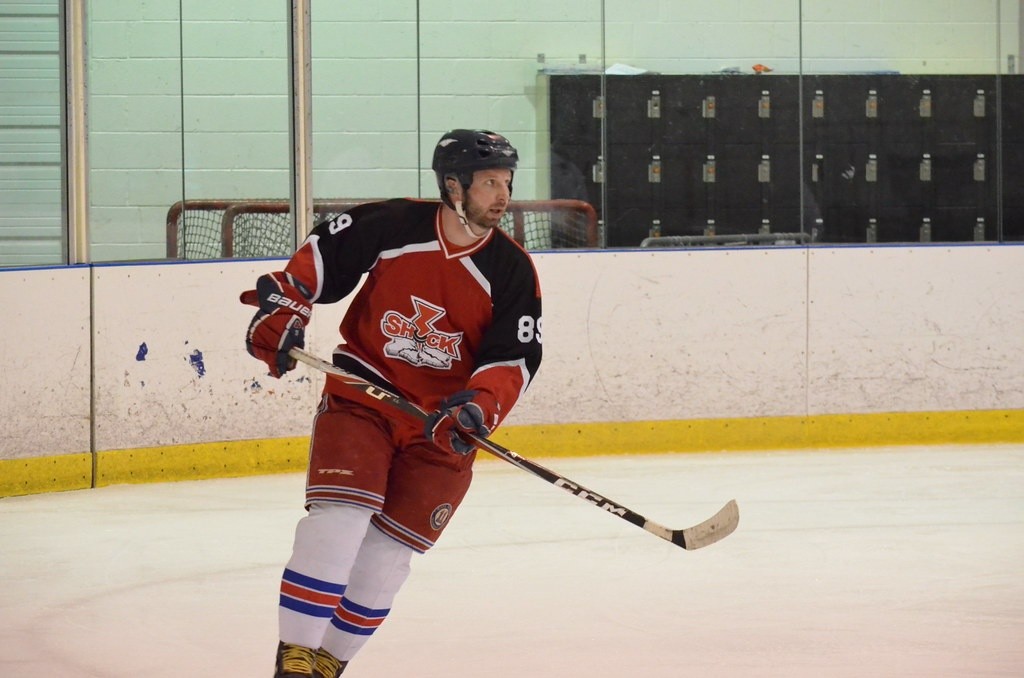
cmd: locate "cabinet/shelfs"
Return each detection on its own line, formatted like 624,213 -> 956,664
551,76 -> 1023,247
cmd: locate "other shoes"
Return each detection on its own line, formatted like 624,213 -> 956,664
312,646 -> 348,678
275,642 -> 318,678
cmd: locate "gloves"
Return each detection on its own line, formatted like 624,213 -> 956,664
426,391 -> 500,455
247,273 -> 311,378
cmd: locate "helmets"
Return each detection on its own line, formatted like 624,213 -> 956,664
432,128 -> 519,211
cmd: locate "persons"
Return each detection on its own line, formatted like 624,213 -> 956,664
240,125 -> 545,677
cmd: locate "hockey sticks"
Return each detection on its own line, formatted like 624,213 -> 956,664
288,347 -> 741,554
